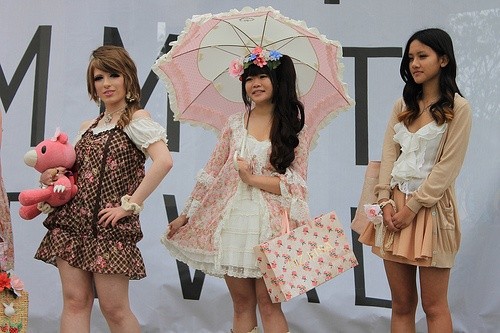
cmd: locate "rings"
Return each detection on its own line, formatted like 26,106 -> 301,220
386,225 -> 388,228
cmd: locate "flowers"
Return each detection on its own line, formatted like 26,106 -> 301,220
228,45 -> 283,80
0,270 -> 26,297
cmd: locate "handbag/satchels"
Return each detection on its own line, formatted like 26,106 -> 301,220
351,158 -> 381,235
253,209 -> 359,304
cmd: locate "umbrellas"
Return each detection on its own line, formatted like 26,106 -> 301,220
150,6 -> 352,171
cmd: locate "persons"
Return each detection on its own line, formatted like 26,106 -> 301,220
18,45 -> 174,333
0,111 -> 14,271
160,47 -> 360,333
358,29 -> 473,333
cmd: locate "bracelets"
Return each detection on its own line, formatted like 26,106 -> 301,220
121,194 -> 144,214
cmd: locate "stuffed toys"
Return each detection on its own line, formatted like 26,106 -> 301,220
18,130 -> 78,220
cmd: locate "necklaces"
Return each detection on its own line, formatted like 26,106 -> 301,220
104,103 -> 128,124
